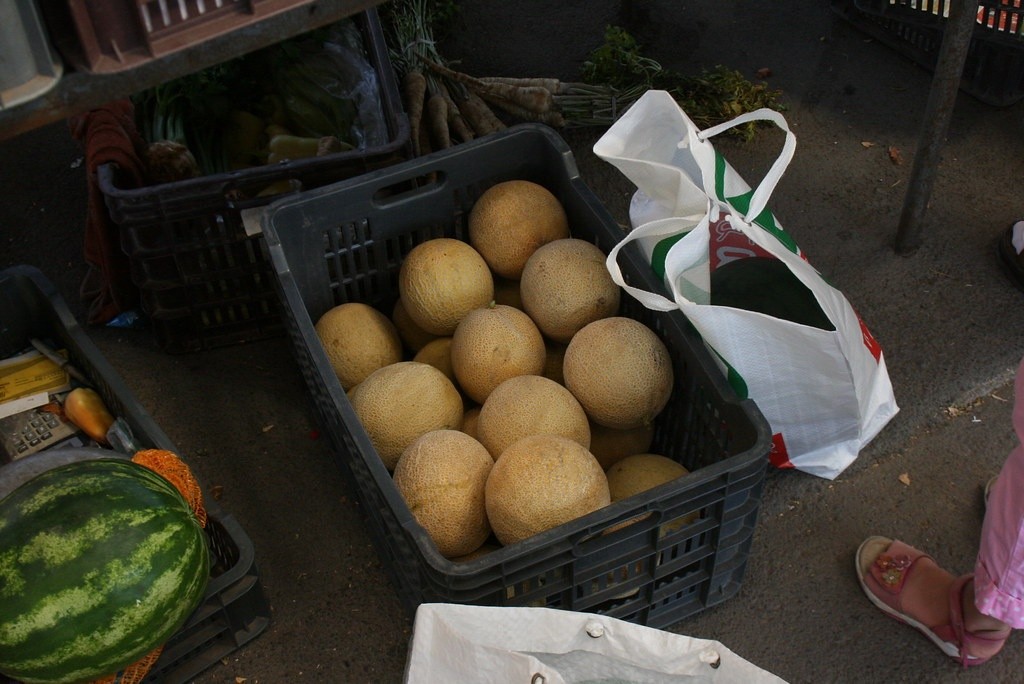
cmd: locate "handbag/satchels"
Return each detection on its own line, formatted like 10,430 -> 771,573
405,603 -> 787,684
594,89 -> 900,481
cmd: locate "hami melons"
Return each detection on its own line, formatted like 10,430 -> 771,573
311,177 -> 699,608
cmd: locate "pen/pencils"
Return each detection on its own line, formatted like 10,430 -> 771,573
31,338 -> 99,390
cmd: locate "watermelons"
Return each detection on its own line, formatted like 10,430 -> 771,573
0,459 -> 208,684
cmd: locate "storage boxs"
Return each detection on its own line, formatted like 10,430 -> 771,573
0,2 -> 774,684
826,0 -> 1024,106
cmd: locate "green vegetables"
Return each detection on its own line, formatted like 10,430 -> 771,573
116,28 -> 373,201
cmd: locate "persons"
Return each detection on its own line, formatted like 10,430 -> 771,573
856,355 -> 1024,667
997,218 -> 1024,292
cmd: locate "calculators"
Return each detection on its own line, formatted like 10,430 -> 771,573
0,395 -> 81,461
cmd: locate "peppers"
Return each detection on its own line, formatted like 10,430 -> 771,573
63,387 -> 118,444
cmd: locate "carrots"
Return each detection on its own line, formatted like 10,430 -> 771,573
398,54 -> 565,166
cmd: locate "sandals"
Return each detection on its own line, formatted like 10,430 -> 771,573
855,536 -> 1011,669
